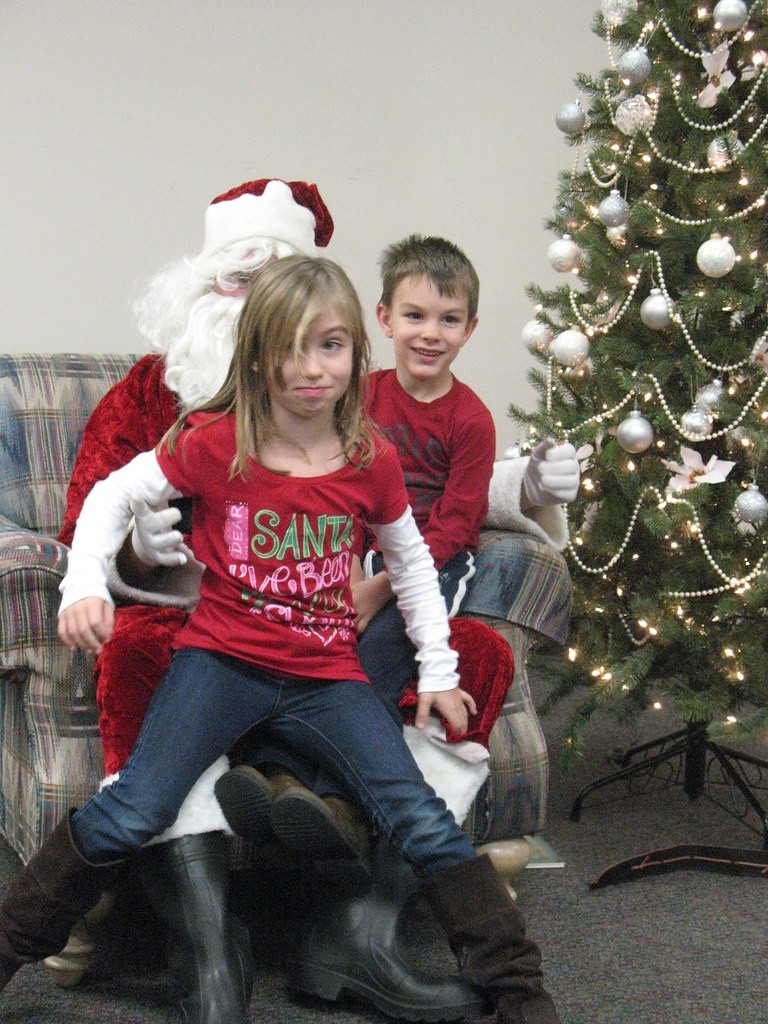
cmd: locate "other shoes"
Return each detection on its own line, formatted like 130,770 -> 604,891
215,765 -> 292,870
270,793 -> 371,891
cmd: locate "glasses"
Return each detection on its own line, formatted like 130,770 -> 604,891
216,273 -> 257,291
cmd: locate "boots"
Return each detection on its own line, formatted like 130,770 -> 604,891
0,807 -> 129,992
423,854 -> 560,1024
290,833 -> 482,1021
138,832 -> 251,1024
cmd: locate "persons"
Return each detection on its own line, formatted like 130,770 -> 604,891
57,179 -> 515,1024
216,237 -> 495,885
0,254 -> 560,1024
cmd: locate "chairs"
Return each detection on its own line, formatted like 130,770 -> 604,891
0,350 -> 577,988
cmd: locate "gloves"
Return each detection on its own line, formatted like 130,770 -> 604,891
523,432 -> 581,506
127,495 -> 188,566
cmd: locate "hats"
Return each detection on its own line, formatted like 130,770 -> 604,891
204,178 -> 332,257
